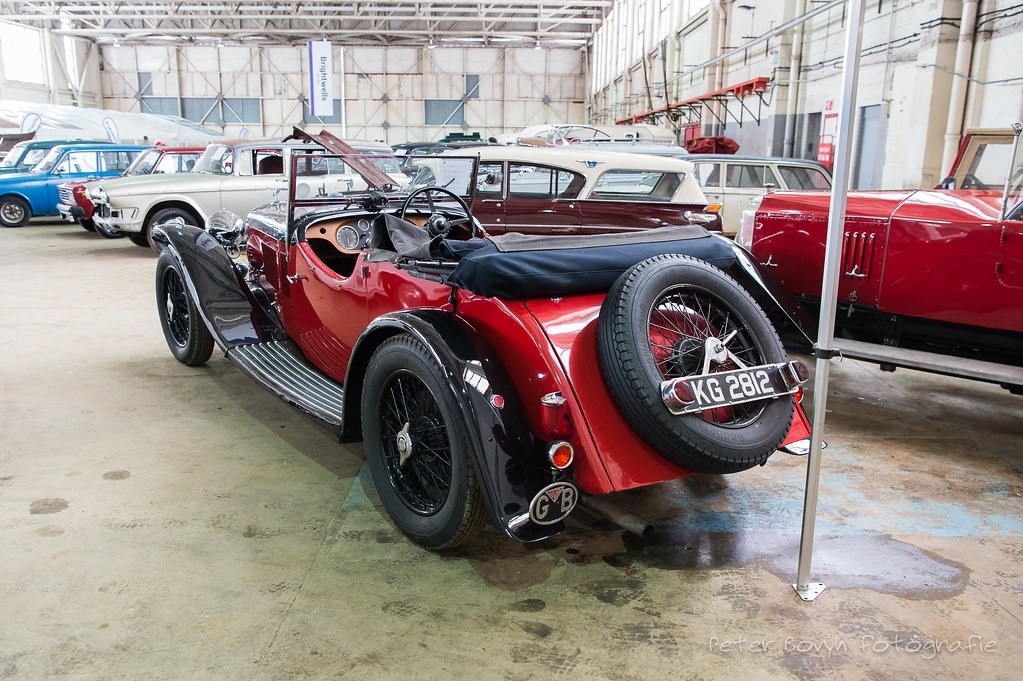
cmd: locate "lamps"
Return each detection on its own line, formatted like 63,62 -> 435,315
534,42 -> 542,50
428,39 -> 435,49
113,36 -> 121,47
217,39 -> 224,48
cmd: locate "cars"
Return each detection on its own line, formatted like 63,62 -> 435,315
735,118 -> 1023,402
0,120 -> 832,256
147,129 -> 829,551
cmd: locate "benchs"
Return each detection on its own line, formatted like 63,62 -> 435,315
448,224 -> 733,301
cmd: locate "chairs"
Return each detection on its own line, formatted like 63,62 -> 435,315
186,160 -> 196,173
259,155 -> 283,175
